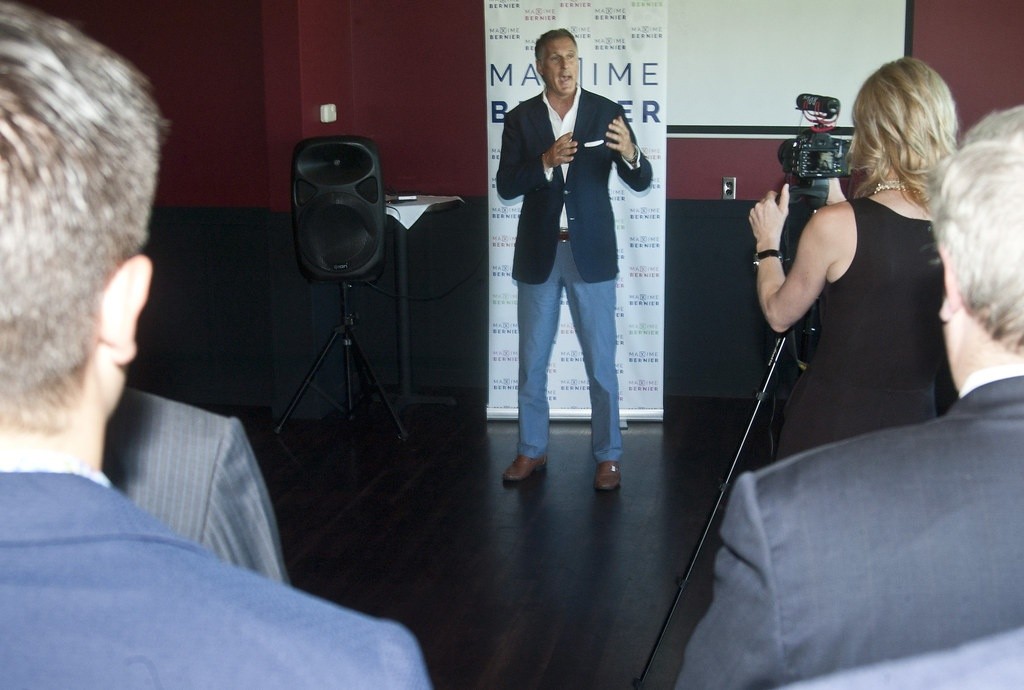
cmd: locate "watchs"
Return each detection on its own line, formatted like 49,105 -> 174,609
753,249 -> 783,272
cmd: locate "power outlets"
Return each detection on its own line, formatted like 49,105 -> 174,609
721,177 -> 737,200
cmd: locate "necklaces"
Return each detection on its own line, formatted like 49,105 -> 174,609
874,181 -> 920,194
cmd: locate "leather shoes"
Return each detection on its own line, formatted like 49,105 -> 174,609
502,452 -> 545,481
594,460 -> 621,491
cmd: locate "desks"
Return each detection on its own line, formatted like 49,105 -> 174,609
322,192 -> 469,406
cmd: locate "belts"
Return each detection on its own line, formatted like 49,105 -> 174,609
558,227 -> 570,241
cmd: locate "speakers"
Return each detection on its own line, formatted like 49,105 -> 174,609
288,133 -> 386,286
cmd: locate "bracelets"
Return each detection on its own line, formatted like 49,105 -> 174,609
625,150 -> 638,164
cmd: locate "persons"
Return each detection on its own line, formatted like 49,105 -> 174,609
747,58 -> 958,462
101,389 -> 289,585
0,5 -> 432,690
675,103 -> 1024,689
495,28 -> 653,491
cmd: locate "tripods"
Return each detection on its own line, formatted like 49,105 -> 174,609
273,280 -> 408,442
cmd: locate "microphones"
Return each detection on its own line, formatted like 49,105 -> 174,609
797,94 -> 840,115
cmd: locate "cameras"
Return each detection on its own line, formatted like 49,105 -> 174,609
777,128 -> 852,178
